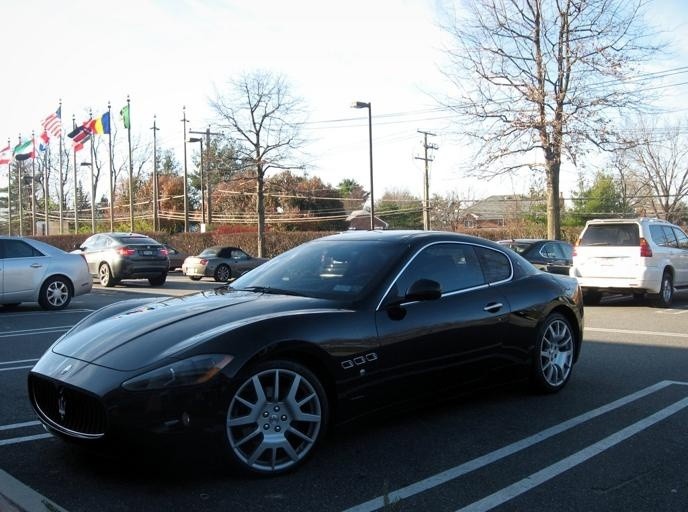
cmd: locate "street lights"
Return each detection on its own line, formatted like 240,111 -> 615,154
19,174 -> 35,236
79,161 -> 96,234
348,99 -> 374,230
182,136 -> 207,234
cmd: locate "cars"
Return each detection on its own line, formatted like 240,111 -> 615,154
0,226 -> 267,311
455,236 -> 575,276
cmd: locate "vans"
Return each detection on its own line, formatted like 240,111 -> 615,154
567,215 -> 687,308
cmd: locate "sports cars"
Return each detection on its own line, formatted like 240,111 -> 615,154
15,225 -> 593,484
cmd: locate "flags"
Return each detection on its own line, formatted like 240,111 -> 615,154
119,104 -> 130,129
72,122 -> 84,153
38,132 -> 50,152
41,105 -> 62,139
66,118 -> 93,145
14,138 -> 36,162
12,141 -> 22,158
83,112 -> 111,135
0,144 -> 11,166
